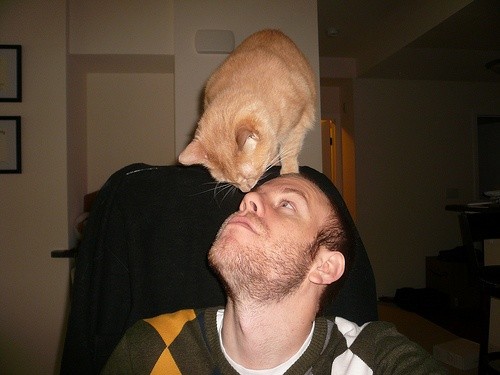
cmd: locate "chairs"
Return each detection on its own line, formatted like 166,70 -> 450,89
58,163 -> 379,375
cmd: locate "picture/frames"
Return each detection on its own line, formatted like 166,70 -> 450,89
0,115 -> 22,174
0,44 -> 23,102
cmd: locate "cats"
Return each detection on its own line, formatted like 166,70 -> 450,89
178,29 -> 316,211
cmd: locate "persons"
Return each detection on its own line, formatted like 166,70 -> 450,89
99,173 -> 445,375
